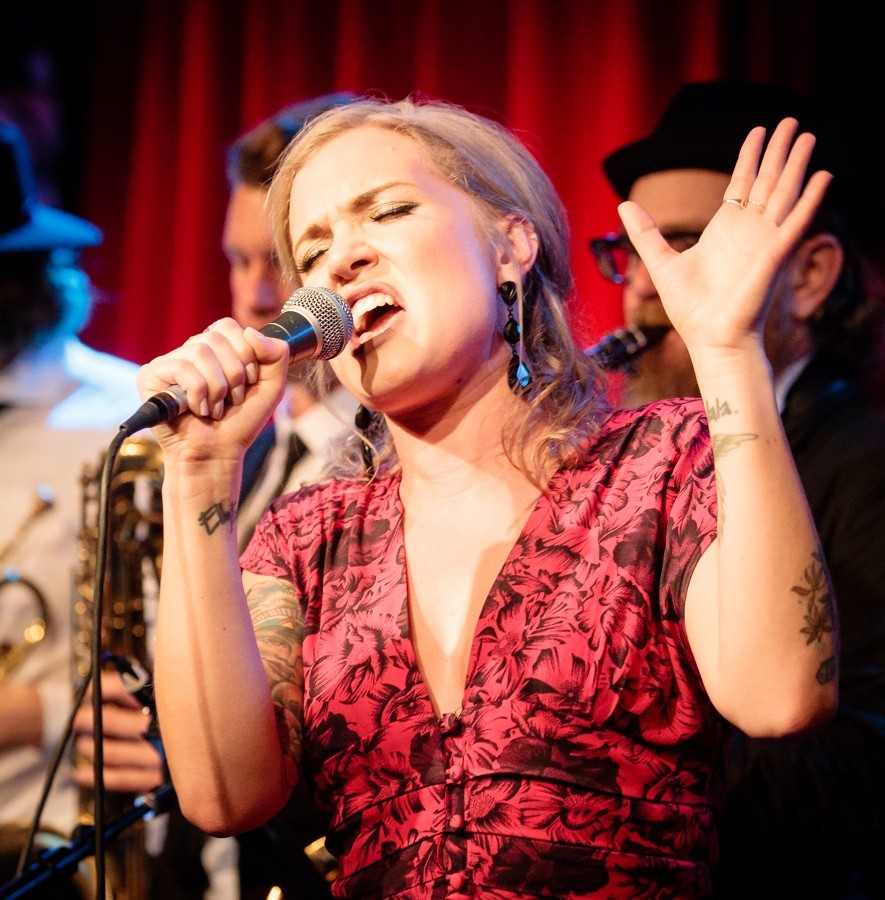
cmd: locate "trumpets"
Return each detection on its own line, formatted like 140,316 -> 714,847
0,486 -> 54,682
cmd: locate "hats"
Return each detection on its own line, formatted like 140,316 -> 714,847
602,81 -> 807,201
0,122 -> 102,251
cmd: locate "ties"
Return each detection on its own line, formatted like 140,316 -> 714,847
239,432 -> 309,560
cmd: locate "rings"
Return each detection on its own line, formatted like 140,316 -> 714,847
748,199 -> 766,211
722,198 -> 747,210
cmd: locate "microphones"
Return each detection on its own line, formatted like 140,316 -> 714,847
112,655 -> 157,715
117,287 -> 353,436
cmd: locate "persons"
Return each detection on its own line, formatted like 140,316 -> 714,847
0,75 -> 884,900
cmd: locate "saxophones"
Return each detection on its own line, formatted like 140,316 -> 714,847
69,433 -> 161,900
581,323 -> 670,371
265,822 -> 356,900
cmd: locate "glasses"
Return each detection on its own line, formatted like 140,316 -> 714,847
590,232 -> 701,284
